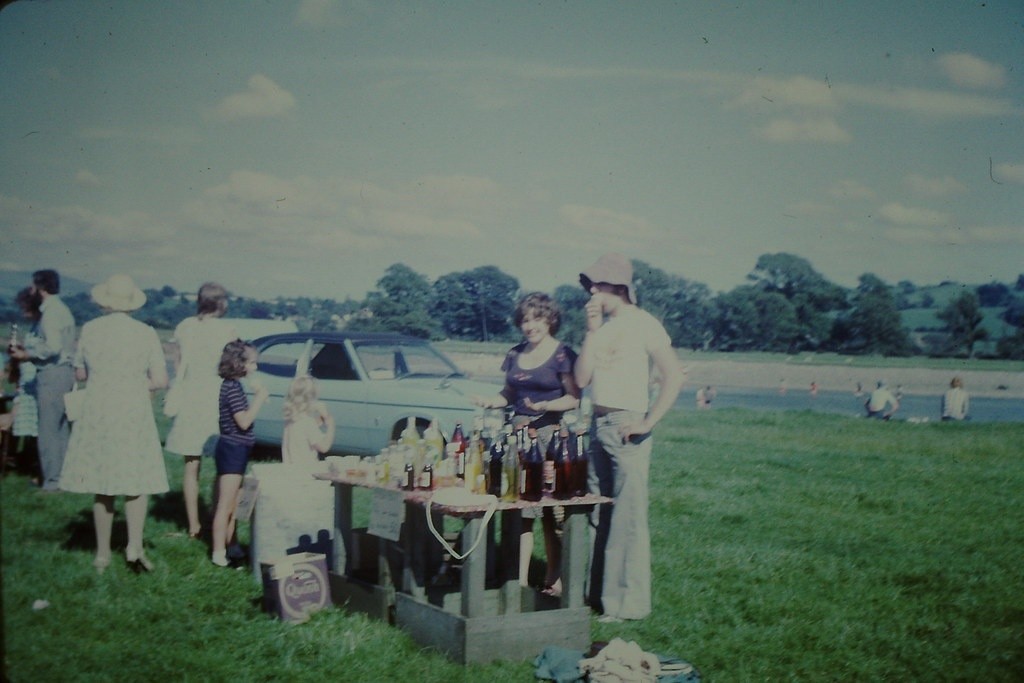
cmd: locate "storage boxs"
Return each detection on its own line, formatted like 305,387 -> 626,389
259,551 -> 330,622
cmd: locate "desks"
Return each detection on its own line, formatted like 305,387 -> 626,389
312,474 -> 611,664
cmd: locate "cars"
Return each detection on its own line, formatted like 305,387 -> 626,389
235,330 -> 597,469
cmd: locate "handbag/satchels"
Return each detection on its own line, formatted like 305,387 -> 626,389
63,380 -> 89,421
163,374 -> 186,419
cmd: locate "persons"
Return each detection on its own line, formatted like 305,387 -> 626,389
942,376 -> 972,421
57,272 -> 171,571
162,281 -> 241,537
0,269 -> 77,491
574,253 -> 680,623
282,374 -> 336,476
810,382 -> 817,394
211,337 -> 270,569
696,385 -> 717,408
864,378 -> 899,420
472,293 -> 583,598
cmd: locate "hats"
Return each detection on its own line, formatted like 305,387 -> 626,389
91,275 -> 147,311
579,253 -> 637,304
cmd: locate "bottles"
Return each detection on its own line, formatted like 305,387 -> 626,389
9,323 -> 17,352
361,418 -> 586,501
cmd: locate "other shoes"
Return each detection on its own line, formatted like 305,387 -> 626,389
125,546 -> 153,571
539,569 -> 567,597
597,613 -> 626,624
212,559 -> 247,571
93,557 -> 113,575
224,546 -> 248,558
188,527 -> 215,539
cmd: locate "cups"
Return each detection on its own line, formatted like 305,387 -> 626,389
323,455 -> 361,482
